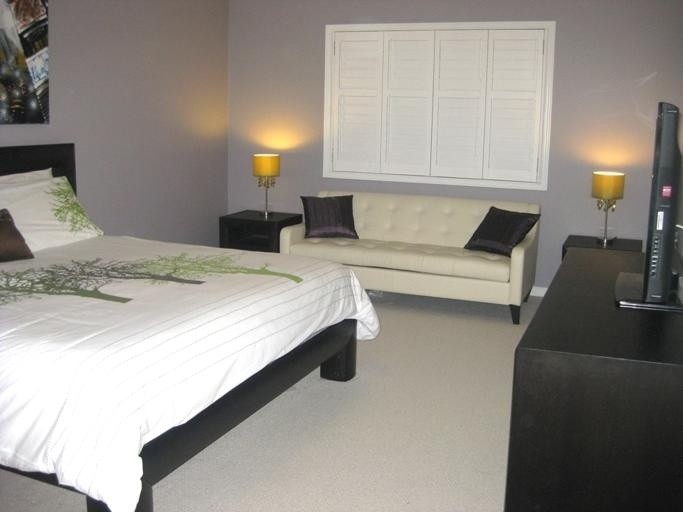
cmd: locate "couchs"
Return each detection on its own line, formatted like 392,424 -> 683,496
279,191 -> 541,326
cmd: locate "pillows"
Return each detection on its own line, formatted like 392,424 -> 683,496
0,167 -> 105,263
298,194 -> 359,240
462,206 -> 540,257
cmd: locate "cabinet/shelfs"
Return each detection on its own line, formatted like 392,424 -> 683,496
561,235 -> 641,261
505,248 -> 681,511
219,210 -> 301,259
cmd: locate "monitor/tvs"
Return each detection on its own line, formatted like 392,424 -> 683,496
614,101 -> 683,312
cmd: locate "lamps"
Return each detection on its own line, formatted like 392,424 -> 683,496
590,171 -> 624,244
252,154 -> 280,216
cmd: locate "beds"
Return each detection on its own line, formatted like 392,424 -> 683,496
0,143 -> 356,511
